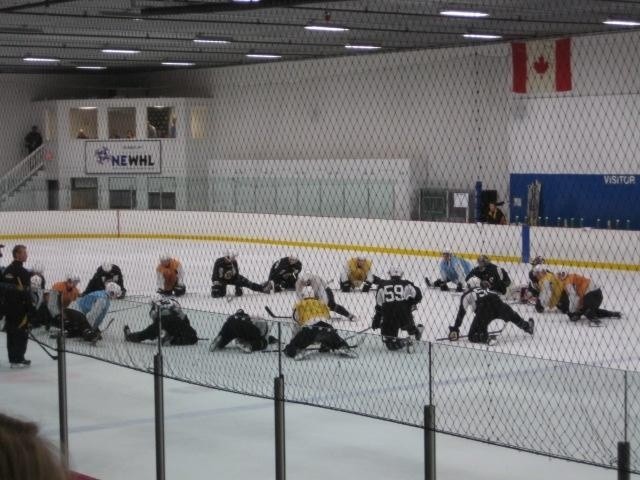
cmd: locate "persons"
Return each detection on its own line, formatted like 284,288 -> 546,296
448,277 -> 535,346
481,203 -> 507,227
210,308 -> 279,353
127,131 -> 136,139
147,121 -> 161,138
108,129 -> 120,139
1,245 -> 36,369
371,264 -> 424,353
507,256 -> 623,327
340,253 -> 383,292
29,259 -> 126,343
169,116 -> 178,137
156,251 -> 186,297
424,250 -> 511,296
269,253 -> 302,293
284,287 -> 358,359
210,250 -> 272,298
0,412 -> 62,480
122,289 -> 198,347
23,126 -> 42,155
77,128 -> 91,140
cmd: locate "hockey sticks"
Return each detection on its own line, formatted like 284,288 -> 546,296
436,323 -> 509,341
101,317 -> 114,332
265,306 -> 291,319
261,333 -> 368,353
424,277 -> 469,290
27,327 -> 60,360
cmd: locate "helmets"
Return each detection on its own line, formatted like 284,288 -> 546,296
30,262 -> 44,286
66,272 -> 81,282
466,277 -> 483,289
389,263 -> 403,279
442,248 -> 453,259
105,281 -> 120,299
530,254 -> 567,280
148,293 -> 163,306
102,260 -> 112,273
223,247 -> 240,260
477,253 -> 490,270
302,286 -> 316,299
355,252 -> 368,261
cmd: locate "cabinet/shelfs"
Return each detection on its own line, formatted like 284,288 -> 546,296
408,187 -> 497,223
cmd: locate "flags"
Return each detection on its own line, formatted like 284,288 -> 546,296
509,39 -> 571,94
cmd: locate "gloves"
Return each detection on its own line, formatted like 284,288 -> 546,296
371,314 -> 383,331
449,325 -> 460,342
174,284 -> 245,299
535,298 -> 582,320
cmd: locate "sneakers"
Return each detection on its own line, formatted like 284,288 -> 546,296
588,311 -> 621,323
83,328 -> 100,342
526,317 -> 535,333
209,335 -> 252,352
124,324 -> 132,341
406,324 -> 425,354
10,359 -> 31,365
263,281 -> 272,295
339,345 -> 357,358
486,335 -> 498,345
